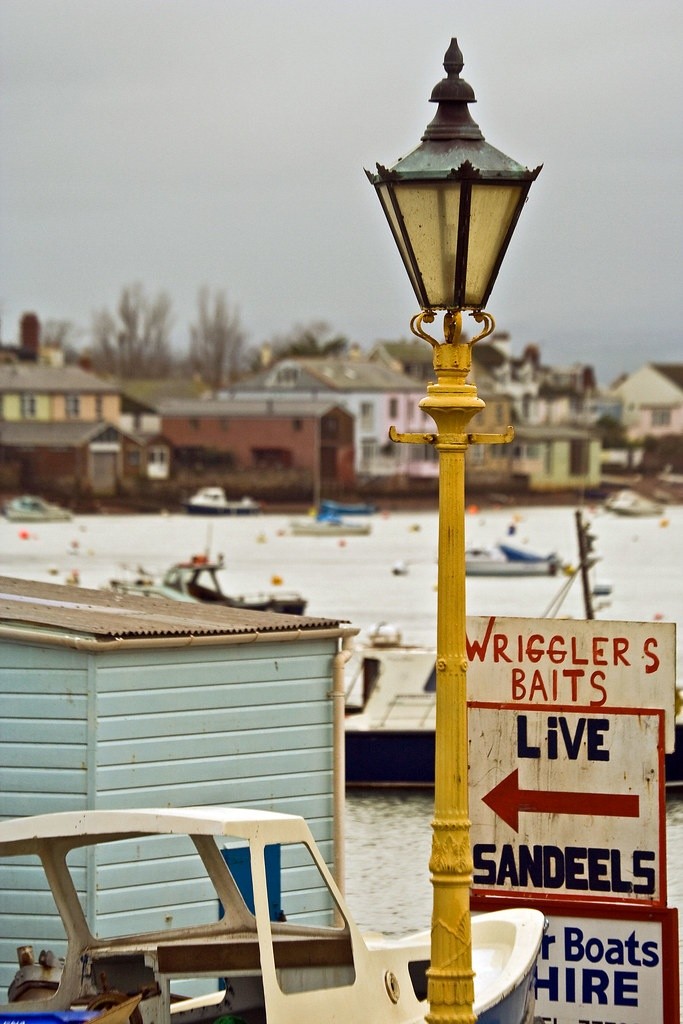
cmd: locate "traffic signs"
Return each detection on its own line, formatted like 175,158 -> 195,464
465,616 -> 680,918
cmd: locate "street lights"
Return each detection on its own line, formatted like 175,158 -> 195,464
360,39 -> 543,1024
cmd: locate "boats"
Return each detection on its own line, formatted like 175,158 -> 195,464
140,528 -> 306,619
341,510 -> 682,792
0,806 -> 547,1024
179,486 -> 261,512
312,497 -> 381,532
605,488 -> 664,518
2,495 -> 75,521
462,526 -> 558,575
